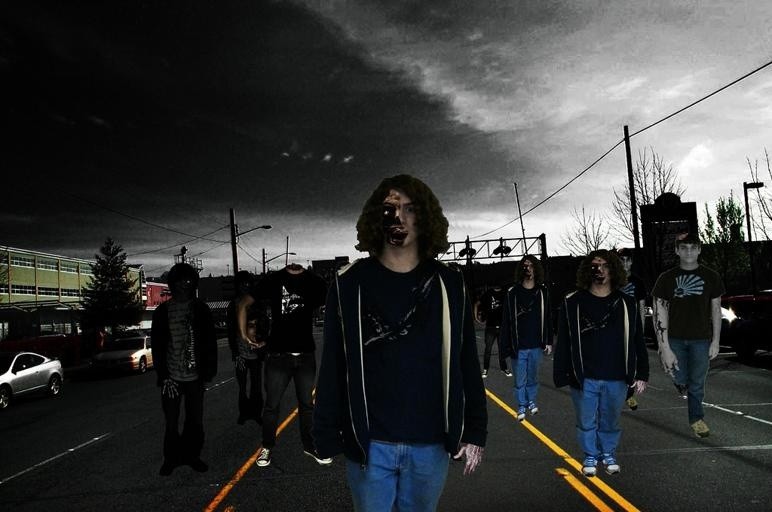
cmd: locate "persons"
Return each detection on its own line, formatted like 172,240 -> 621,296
315,173 -> 488,511
474,231 -> 727,476
150,262 -> 333,473
